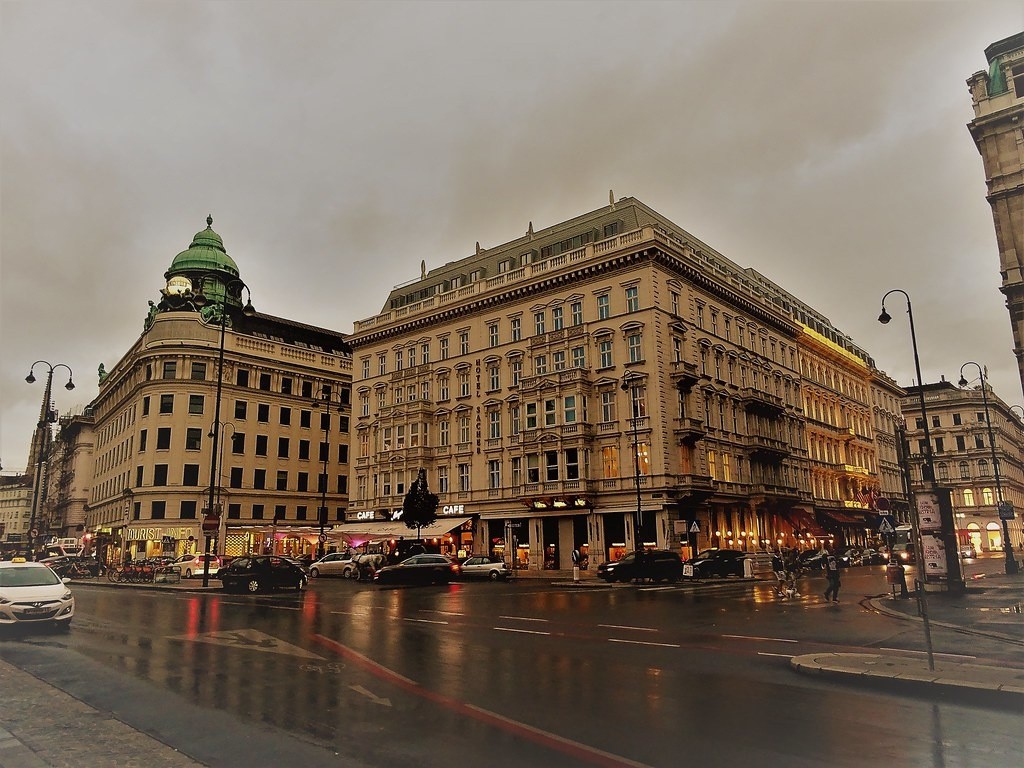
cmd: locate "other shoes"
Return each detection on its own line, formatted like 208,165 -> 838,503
795,593 -> 801,597
778,591 -> 783,596
833,599 -> 839,603
822,593 -> 828,602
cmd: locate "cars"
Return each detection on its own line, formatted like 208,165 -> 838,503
216,555 -> 310,594
957,545 -> 978,560
372,553 -> 462,585
687,548 -> 746,577
173,553 -> 220,579
309,551 -> 385,580
462,554 -> 510,579
0,558 -> 76,632
785,541 -> 922,573
142,555 -> 176,572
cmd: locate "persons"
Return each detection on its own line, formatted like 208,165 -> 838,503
1019,542 -> 1023,551
396,536 -> 406,556
122,550 -> 132,569
340,546 -> 362,557
290,549 -> 292,557
823,544 -> 840,604
0,548 -> 45,562
383,549 -> 386,555
490,551 -> 505,562
267,543 -> 273,554
766,545 -> 803,601
544,554 -> 555,570
579,554 -> 589,570
298,546 -> 302,554
466,553 -> 472,560
444,549 -> 451,556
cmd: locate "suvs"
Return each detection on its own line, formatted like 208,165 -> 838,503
597,549 -> 683,583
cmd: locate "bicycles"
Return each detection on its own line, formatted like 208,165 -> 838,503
50,557 -> 96,580
108,559 -> 169,585
683,561 -> 701,582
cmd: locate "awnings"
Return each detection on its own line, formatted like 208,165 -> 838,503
778,507 -> 830,541
326,517 -> 473,540
831,511 -> 900,528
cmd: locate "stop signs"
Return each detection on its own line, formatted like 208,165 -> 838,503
200,513 -> 220,534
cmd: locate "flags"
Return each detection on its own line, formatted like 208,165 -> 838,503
855,491 -> 874,507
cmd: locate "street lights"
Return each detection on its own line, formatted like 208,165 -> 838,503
23,360 -> 76,558
622,369 -> 649,585
311,388 -> 347,559
877,289 -> 972,598
197,273 -> 257,585
961,362 -> 1018,575
